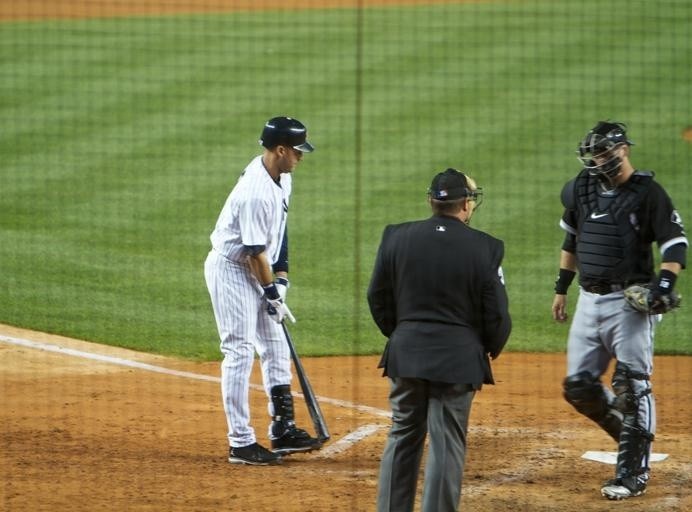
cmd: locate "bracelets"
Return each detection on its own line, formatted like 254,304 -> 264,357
261,281 -> 279,301
552,268 -> 577,296
654,269 -> 677,292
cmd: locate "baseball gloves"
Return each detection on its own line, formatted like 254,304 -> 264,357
623,283 -> 682,315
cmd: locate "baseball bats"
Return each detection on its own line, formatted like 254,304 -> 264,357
281,318 -> 331,445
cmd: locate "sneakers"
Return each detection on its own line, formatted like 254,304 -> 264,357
272,428 -> 322,452
228,442 -> 281,466
601,472 -> 648,500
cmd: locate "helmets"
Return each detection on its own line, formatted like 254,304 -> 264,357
260,116 -> 313,152
575,120 -> 634,190
430,169 -> 483,223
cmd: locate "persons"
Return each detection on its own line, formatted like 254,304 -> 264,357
367,169 -> 513,511
204,116 -> 326,464
549,122 -> 689,499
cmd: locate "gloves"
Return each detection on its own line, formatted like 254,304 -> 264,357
261,277 -> 297,323
